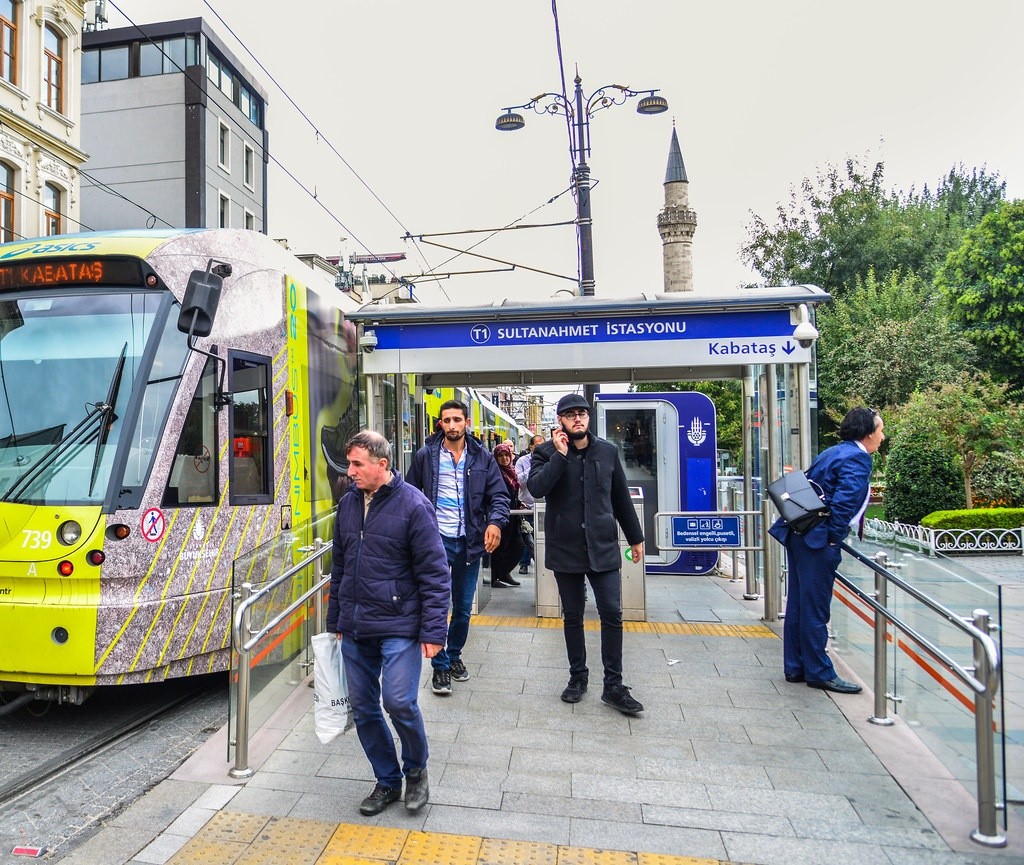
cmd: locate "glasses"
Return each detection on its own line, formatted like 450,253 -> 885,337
869,407 -> 875,417
561,411 -> 589,420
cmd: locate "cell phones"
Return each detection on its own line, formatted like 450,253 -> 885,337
558,427 -> 567,445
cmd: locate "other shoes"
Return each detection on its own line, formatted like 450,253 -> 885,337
499,574 -> 520,585
482,579 -> 490,587
519,565 -> 528,574
491,580 -> 507,588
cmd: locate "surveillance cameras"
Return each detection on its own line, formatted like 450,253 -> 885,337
359,332 -> 378,353
793,323 -> 819,348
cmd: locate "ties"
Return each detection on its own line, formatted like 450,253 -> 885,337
858,512 -> 865,540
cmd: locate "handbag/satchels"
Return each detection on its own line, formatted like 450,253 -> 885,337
765,469 -> 828,536
311,631 -> 357,745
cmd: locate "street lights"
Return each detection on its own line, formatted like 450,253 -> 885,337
495,62 -> 668,415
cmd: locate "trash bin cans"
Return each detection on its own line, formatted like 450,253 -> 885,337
533,486 -> 645,621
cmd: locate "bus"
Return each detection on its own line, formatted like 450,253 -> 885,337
0,226 -> 536,717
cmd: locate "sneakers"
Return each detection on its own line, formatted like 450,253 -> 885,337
601,685 -> 643,712
560,681 -> 587,702
450,659 -> 469,681
432,668 -> 452,693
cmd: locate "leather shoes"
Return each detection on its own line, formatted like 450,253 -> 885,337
360,782 -> 402,814
785,674 -> 806,683
404,767 -> 429,810
807,675 -> 862,693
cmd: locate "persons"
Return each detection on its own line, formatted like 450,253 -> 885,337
403,401 -> 512,692
784,406 -> 885,694
325,431 -> 449,815
490,436 -> 545,587
527,395 -> 645,712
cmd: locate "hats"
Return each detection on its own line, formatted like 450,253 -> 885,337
557,393 -> 590,416
